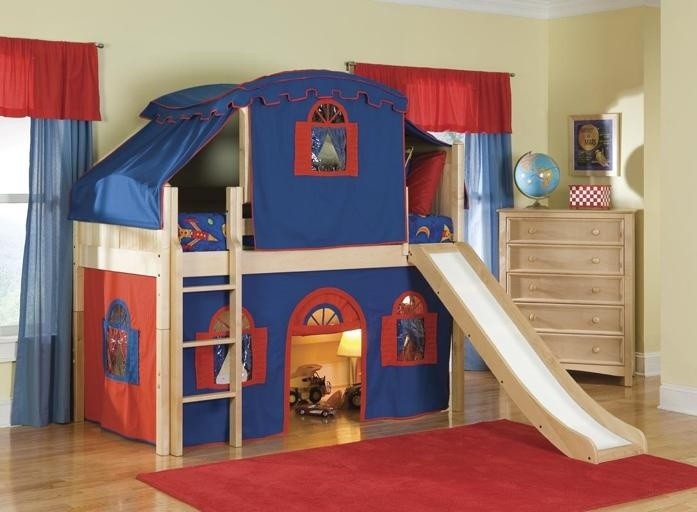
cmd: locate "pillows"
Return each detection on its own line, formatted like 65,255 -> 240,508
402,151 -> 446,216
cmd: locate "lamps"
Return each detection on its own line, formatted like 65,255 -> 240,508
337,329 -> 361,387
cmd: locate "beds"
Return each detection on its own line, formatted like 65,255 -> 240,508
72,67 -> 468,460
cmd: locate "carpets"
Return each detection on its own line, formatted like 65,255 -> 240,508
134,417 -> 697,511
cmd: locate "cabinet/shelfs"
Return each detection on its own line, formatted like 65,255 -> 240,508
493,207 -> 639,387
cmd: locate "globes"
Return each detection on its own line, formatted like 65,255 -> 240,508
512,151 -> 561,209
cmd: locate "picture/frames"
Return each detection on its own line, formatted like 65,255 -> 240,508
565,110 -> 621,180
